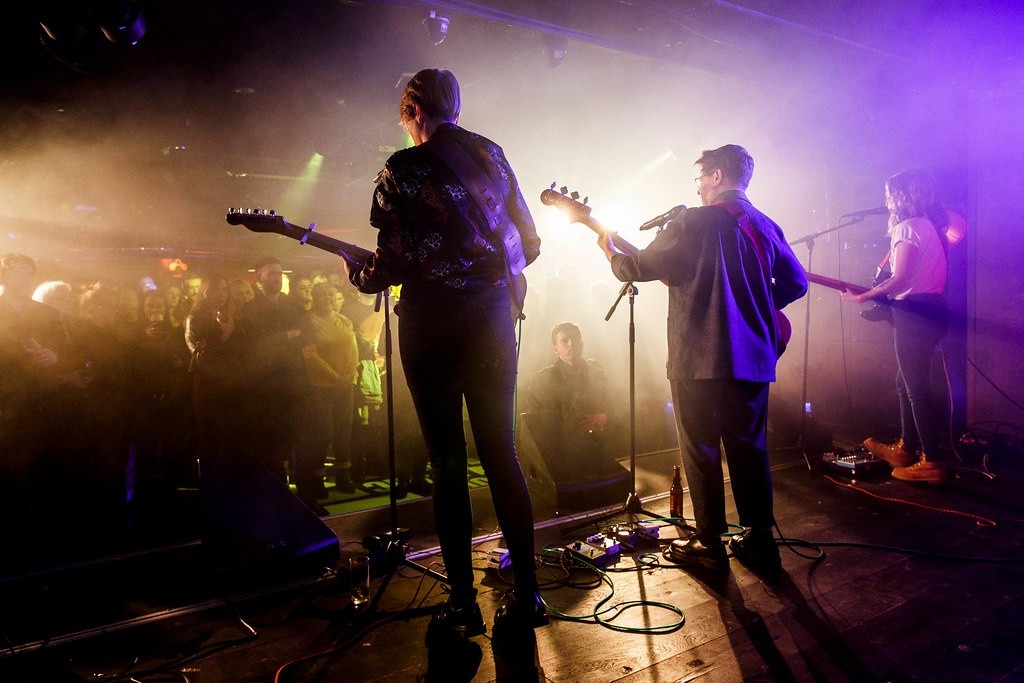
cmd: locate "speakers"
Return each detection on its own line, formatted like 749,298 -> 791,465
196,443 -> 341,571
514,410 -> 633,505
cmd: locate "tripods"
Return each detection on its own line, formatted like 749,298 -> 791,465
358,289 -> 448,628
560,282 -> 696,538
768,283 -> 825,474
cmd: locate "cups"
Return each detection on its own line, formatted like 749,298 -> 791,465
150,314 -> 163,324
336,556 -> 369,609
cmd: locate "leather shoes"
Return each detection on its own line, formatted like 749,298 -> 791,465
727,533 -> 782,569
493,592 -> 549,629
432,600 -> 487,638
864,438 -> 909,468
891,453 -> 947,485
668,534 -> 728,569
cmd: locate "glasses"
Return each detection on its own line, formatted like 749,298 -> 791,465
694,168 -> 714,188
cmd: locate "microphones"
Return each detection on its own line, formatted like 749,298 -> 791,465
849,206 -> 888,216
642,209 -> 679,230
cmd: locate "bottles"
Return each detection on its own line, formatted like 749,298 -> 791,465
670,465 -> 685,517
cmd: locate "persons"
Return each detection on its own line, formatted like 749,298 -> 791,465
343,67 -> 548,640
597,143 -> 808,571
0,254 -> 430,554
527,322 -> 627,443
839,169 -> 950,482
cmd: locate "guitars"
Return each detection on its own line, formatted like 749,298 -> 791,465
225,206 -> 375,271
540,182 -> 791,349
801,270 -> 892,322
